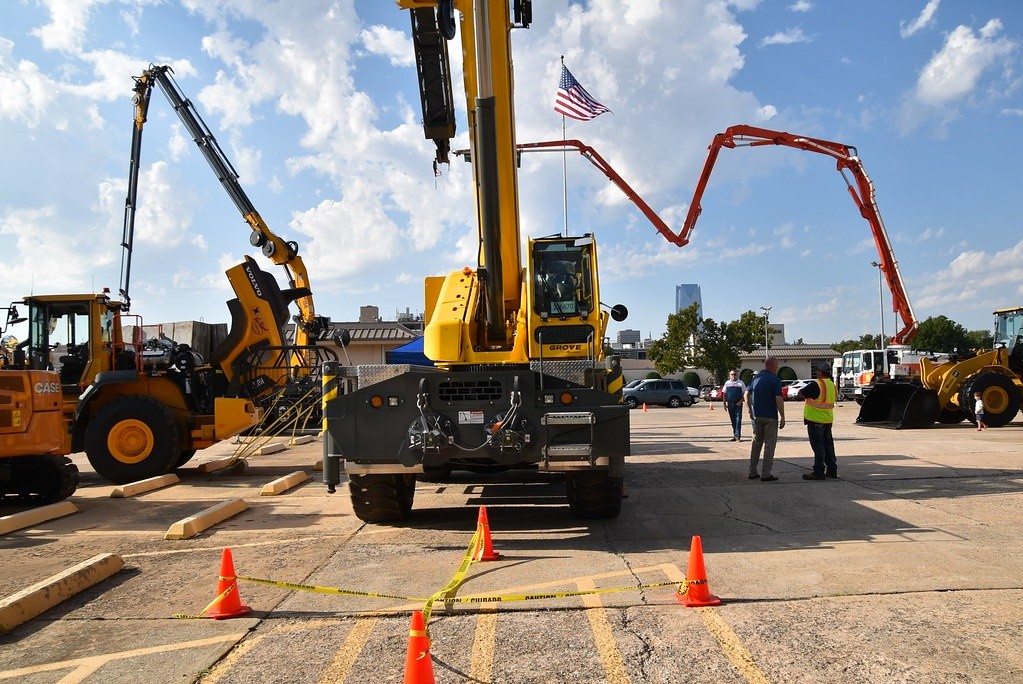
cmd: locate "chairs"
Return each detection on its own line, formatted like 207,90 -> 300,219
59,340 -> 89,363
542,260 -> 576,293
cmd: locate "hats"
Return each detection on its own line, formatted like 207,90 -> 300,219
751,371 -> 759,376
815,362 -> 831,373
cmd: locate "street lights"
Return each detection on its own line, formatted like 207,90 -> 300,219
870,261 -> 885,350
760,305 -> 772,362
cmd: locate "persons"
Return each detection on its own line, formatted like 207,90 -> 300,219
721,369 -> 761,443
797,361 -> 838,480
744,358 -> 786,482
973,391 -> 988,432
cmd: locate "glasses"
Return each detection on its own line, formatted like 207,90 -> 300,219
731,374 -> 736,376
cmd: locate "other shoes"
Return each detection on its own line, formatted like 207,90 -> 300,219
736,439 -> 741,442
761,474 -> 779,481
983,425 -> 987,430
975,430 -> 982,432
803,471 -> 825,480
748,474 -> 760,479
826,471 -> 838,478
730,439 -> 736,441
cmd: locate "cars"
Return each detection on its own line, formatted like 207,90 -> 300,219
621,377 -> 847,409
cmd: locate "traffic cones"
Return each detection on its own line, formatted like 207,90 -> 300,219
205,549 -> 251,620
464,505 -> 502,560
402,610 -> 436,684
642,403 -> 649,413
709,402 -> 715,410
673,534 -> 722,607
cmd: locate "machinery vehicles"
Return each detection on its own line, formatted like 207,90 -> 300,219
852,306 -> 1023,431
0,285 -> 265,483
116,61 -> 352,428
321,0 -> 630,529
0,368 -> 80,504
450,124 -> 967,407
